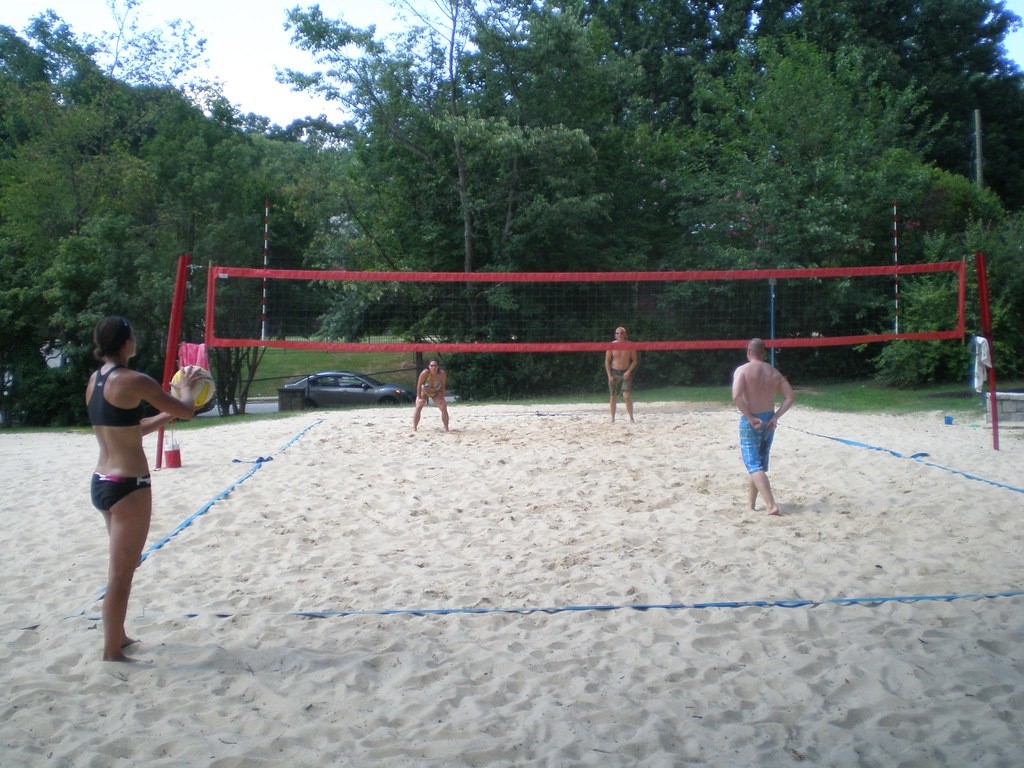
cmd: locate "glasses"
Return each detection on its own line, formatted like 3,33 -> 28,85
429,364 -> 438,367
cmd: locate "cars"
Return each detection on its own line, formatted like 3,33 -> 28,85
285,371 -> 417,408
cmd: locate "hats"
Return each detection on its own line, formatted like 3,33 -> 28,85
616,327 -> 628,338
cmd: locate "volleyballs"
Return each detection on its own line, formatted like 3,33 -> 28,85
170,365 -> 216,410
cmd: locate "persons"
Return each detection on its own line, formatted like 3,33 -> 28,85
733,338 -> 794,514
85,316 -> 205,664
605,327 -> 638,420
414,360 -> 450,431
